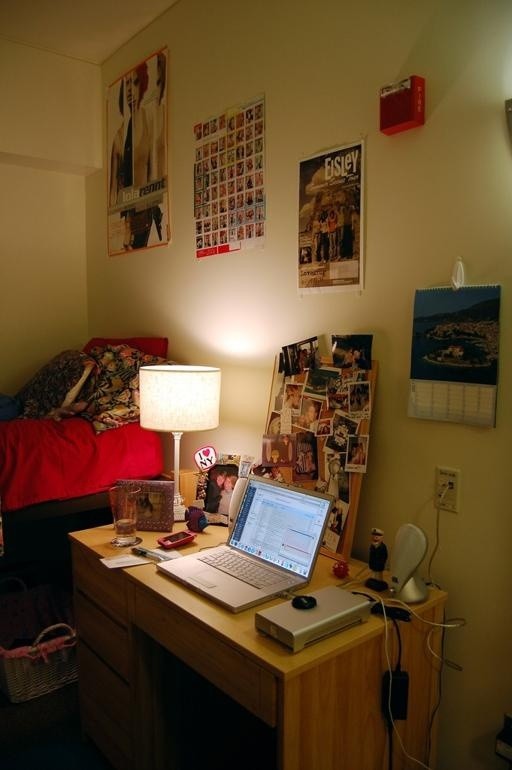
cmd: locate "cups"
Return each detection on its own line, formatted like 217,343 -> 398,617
110,485 -> 142,544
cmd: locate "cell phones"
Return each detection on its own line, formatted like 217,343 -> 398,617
158,530 -> 196,549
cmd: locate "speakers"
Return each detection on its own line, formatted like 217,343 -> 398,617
388,523 -> 428,605
227,478 -> 247,539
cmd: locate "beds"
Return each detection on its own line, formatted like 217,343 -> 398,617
0,338 -> 168,621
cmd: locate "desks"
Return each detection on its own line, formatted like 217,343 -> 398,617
68,517 -> 450,770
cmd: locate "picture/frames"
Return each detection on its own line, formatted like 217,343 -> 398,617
196,455 -> 256,524
116,479 -> 175,532
260,333 -> 378,563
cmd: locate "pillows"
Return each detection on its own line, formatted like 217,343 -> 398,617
12,350 -> 100,419
75,344 -> 177,435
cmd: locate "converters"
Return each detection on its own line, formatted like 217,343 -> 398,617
383,669 -> 408,721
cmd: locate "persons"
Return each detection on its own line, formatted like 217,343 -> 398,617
203,471 -> 228,513
110,53 -> 166,250
218,475 -> 237,516
254,348 -> 366,536
311,199 -> 356,265
365,527 -> 388,592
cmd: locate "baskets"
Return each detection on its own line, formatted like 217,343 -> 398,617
0,575 -> 80,705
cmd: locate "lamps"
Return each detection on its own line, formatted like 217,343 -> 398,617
137,364 -> 219,521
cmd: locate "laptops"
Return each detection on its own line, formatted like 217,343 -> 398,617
155,475 -> 335,615
